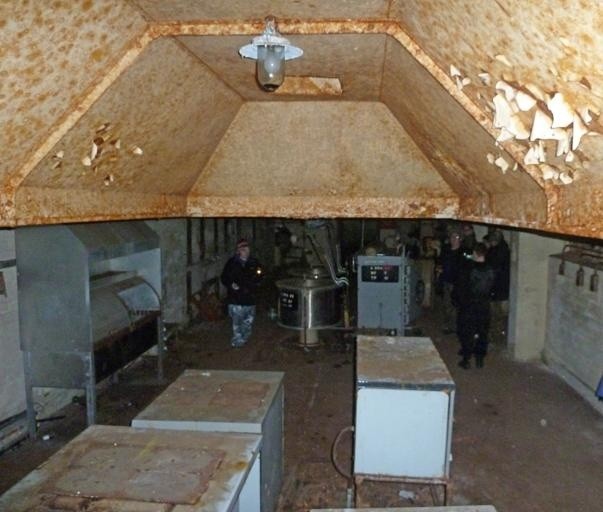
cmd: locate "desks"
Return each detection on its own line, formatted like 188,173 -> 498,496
1,424 -> 264,512
132,369 -> 285,511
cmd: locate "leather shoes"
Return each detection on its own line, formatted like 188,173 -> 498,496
459,360 -> 470,368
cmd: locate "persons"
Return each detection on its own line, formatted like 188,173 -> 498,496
221,238 -> 263,349
406,219 -> 510,372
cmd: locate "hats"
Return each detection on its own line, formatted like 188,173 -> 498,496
236,239 -> 248,249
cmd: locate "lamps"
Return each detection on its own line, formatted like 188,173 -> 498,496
238,16 -> 304,91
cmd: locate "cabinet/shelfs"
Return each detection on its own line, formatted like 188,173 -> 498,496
353,335 -> 456,507
